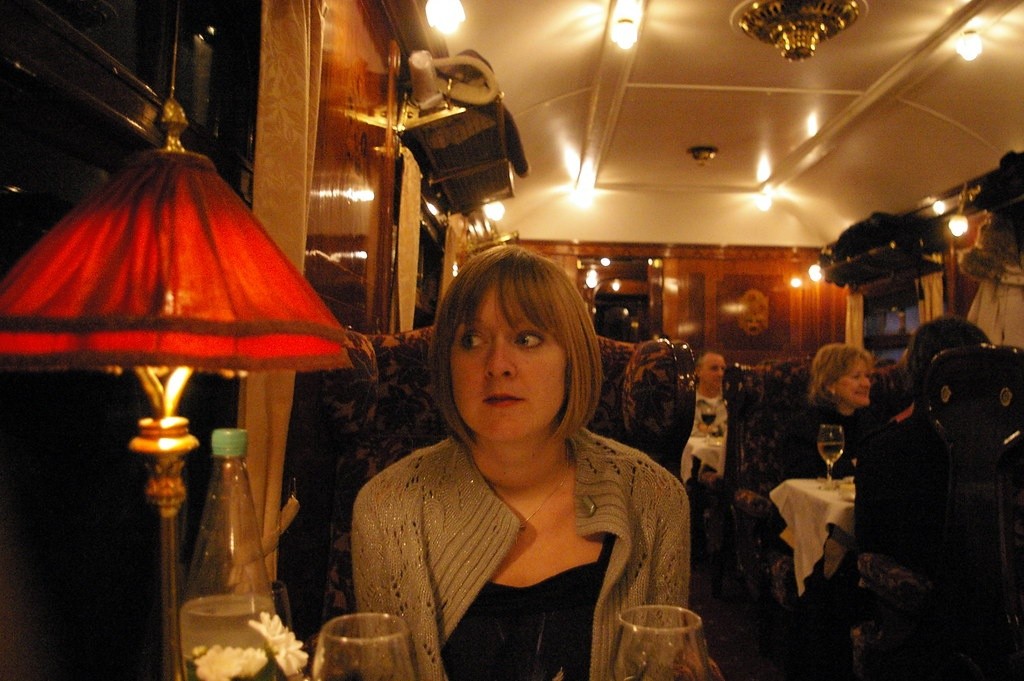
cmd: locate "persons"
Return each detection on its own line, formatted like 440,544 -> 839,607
671,353 -> 738,573
776,317 -> 990,575
350,244 -> 690,681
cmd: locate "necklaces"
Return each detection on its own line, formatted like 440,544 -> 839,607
520,444 -> 569,531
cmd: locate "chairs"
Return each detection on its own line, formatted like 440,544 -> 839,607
848,338 -> 1024,681
311,331 -> 696,630
721,346 -> 931,623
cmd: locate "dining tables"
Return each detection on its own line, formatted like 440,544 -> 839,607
766,469 -> 858,660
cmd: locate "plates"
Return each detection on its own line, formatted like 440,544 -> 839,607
711,441 -> 725,447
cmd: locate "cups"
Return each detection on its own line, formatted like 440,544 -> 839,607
607,605 -> 710,681
310,613 -> 418,681
266,578 -> 295,680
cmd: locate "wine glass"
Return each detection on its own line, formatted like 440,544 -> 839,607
700,406 -> 717,443
815,424 -> 846,490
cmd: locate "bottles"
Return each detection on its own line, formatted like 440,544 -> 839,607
180,425 -> 276,681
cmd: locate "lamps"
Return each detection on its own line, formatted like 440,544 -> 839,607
725,0 -> 869,64
0,99 -> 357,681
686,147 -> 719,167
